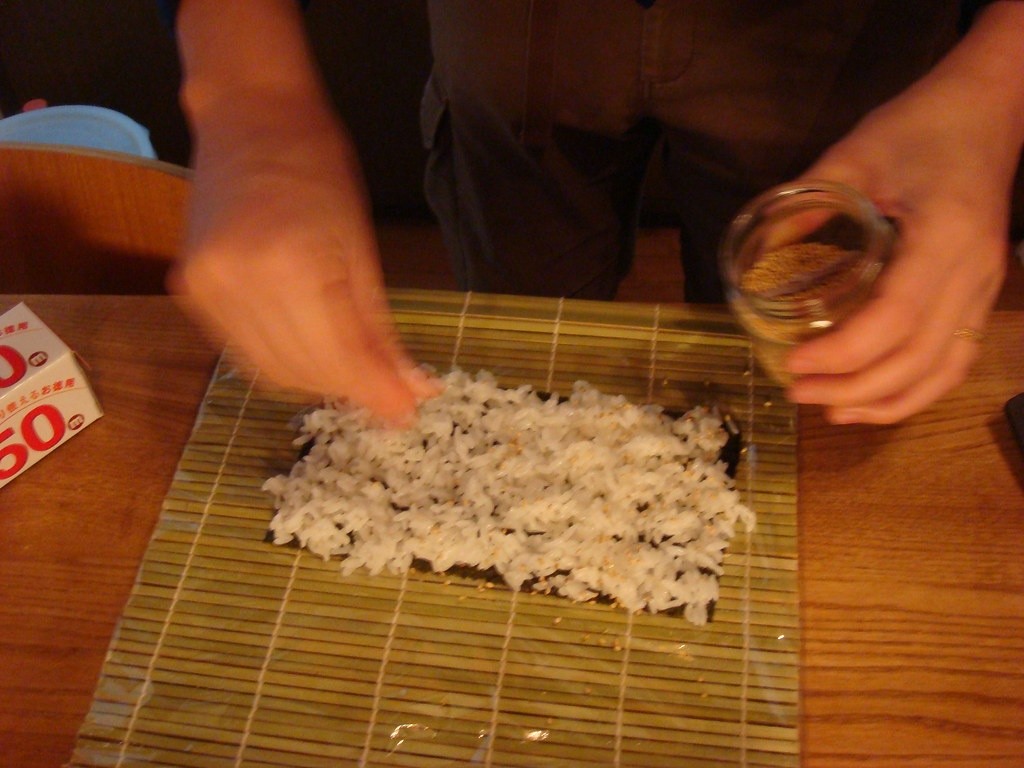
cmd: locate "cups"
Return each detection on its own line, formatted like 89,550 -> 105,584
718,177 -> 899,391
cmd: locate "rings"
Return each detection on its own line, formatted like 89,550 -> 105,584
956,327 -> 986,343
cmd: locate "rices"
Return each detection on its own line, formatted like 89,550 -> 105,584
261,364 -> 756,628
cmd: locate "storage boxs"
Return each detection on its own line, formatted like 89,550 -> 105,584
0,301 -> 104,492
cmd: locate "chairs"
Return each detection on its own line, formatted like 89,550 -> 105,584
0,141 -> 194,292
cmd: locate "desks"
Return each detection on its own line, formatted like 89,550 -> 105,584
1,292 -> 1024,767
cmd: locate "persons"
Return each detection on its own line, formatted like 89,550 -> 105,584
173,0 -> 1024,424
